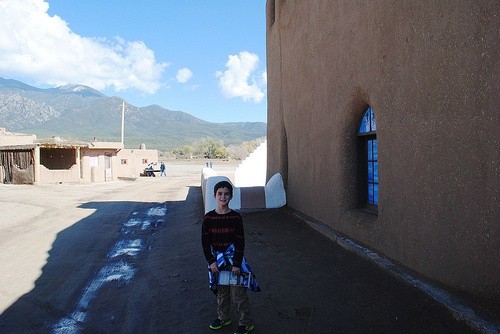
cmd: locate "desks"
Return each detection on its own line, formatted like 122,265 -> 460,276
145,169 -> 161,173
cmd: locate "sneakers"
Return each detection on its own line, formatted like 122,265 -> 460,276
209,318 -> 231,329
234,322 -> 254,334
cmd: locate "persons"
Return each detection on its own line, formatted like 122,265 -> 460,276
201,181 -> 255,334
160,161 -> 167,177
147,162 -> 156,177
